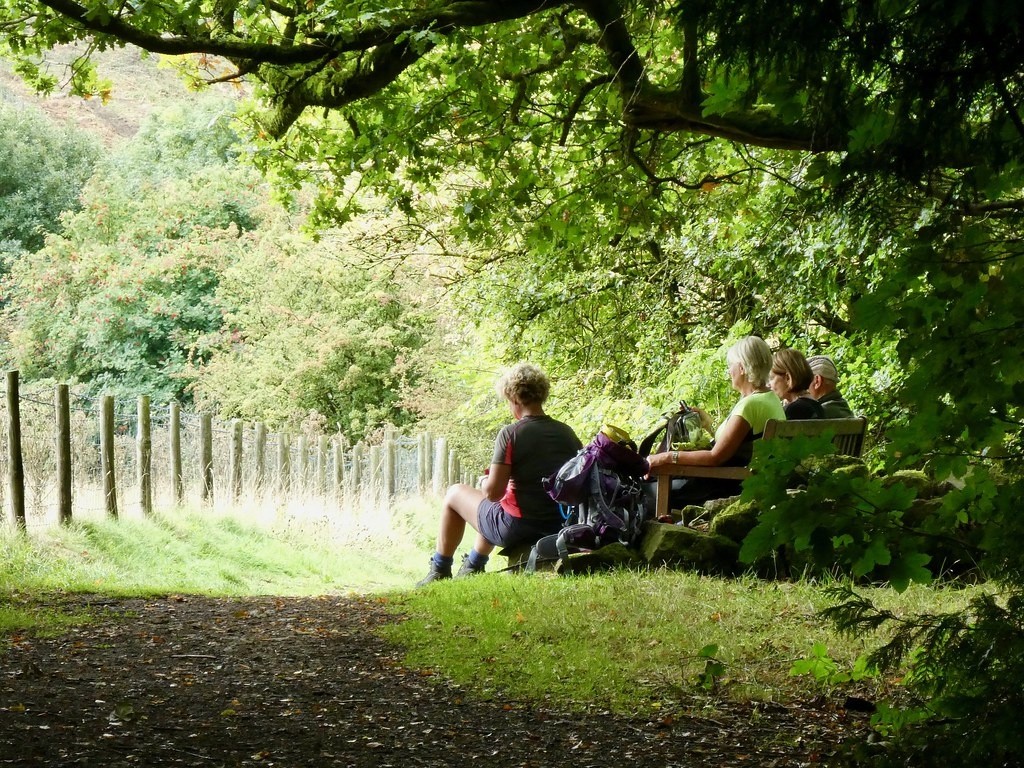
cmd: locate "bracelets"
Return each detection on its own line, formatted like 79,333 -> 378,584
478,474 -> 488,485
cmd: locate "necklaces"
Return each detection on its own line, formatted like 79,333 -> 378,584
521,414 -> 544,418
752,388 -> 760,394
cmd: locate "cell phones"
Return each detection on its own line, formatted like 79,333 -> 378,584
680,400 -> 689,413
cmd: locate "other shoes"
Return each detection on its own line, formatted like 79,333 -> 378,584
454,553 -> 486,578
415,557 -> 453,588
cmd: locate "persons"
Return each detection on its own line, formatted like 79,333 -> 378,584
416,362 -> 582,587
643,335 -> 787,513
766,349 -> 855,420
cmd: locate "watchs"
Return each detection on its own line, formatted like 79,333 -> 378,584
672,451 -> 679,464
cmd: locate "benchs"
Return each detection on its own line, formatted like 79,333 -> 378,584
649,416 -> 869,514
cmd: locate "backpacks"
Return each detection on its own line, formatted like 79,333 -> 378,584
635,410 -> 702,484
522,459 -> 652,576
